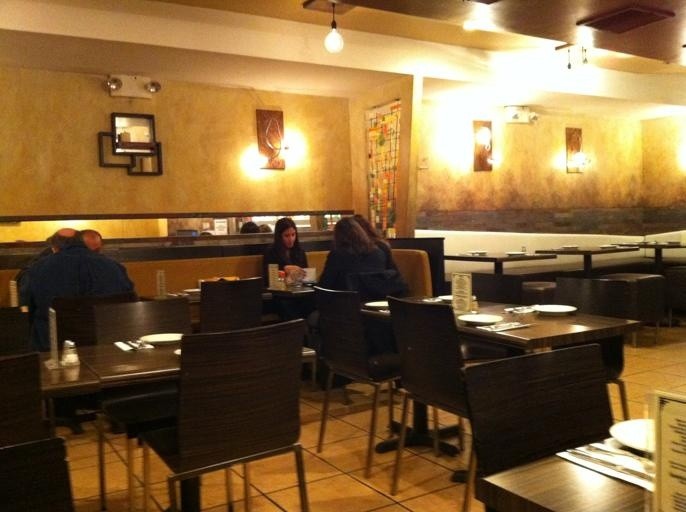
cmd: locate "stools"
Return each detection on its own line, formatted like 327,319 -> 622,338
663,267 -> 686,328
555,273 -> 666,347
523,283 -> 556,305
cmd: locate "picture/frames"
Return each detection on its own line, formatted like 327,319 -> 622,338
111,111 -> 158,155
128,141 -> 163,176
98,131 -> 137,167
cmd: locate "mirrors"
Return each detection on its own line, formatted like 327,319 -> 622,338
0,208 -> 355,239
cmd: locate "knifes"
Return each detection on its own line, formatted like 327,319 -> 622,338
567,449 -> 656,485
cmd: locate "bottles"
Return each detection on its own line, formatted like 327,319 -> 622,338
521,245 -> 526,252
64,342 -> 80,363
277,271 -> 287,290
61,340 -> 70,362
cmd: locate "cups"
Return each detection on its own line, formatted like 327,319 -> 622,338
300,266 -> 317,282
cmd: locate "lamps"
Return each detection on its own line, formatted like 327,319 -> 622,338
324,3 -> 344,54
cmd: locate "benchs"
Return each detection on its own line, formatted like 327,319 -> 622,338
413,210 -> 656,302
0,240 -> 330,308
643,207 -> 686,267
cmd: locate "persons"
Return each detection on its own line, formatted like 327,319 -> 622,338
264,218 -> 308,288
258,225 -> 272,232
240,221 -> 260,233
353,214 -> 408,298
320,217 -> 387,389
27,230 -> 138,304
25,228 -> 131,349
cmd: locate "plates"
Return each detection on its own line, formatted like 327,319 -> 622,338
467,251 -> 488,255
608,419 -> 654,452
183,288 -> 202,294
174,349 -> 181,356
599,246 -> 616,248
139,333 -> 185,344
531,305 -> 577,314
458,314 -> 503,324
562,245 -> 577,249
364,301 -> 389,309
438,295 -> 477,302
506,252 -> 527,255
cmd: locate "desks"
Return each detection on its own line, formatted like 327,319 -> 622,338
534,247 -> 640,279
610,242 -> 686,273
443,253 -> 558,276
